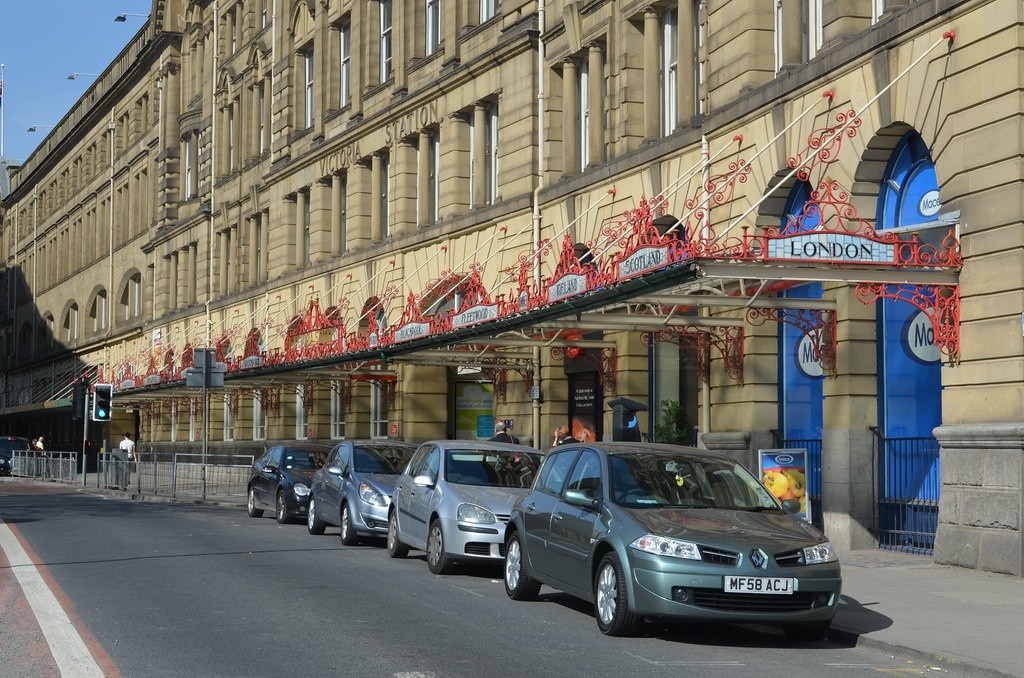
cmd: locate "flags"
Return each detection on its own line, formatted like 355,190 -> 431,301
74,372 -> 94,393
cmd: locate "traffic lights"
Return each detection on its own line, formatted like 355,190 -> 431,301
96,386 -> 111,419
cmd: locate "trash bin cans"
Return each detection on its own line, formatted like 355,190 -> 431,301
109,448 -> 129,487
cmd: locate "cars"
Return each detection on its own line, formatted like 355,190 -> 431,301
306,438 -> 421,546
505,440 -> 835,544
247,444 -> 336,525
387,439 -> 546,576
0,436 -> 31,477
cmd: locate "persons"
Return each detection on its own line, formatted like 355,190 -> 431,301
483,422 -> 520,444
578,424 -> 592,443
553,425 -> 581,447
32,436 -> 44,451
119,432 -> 137,484
